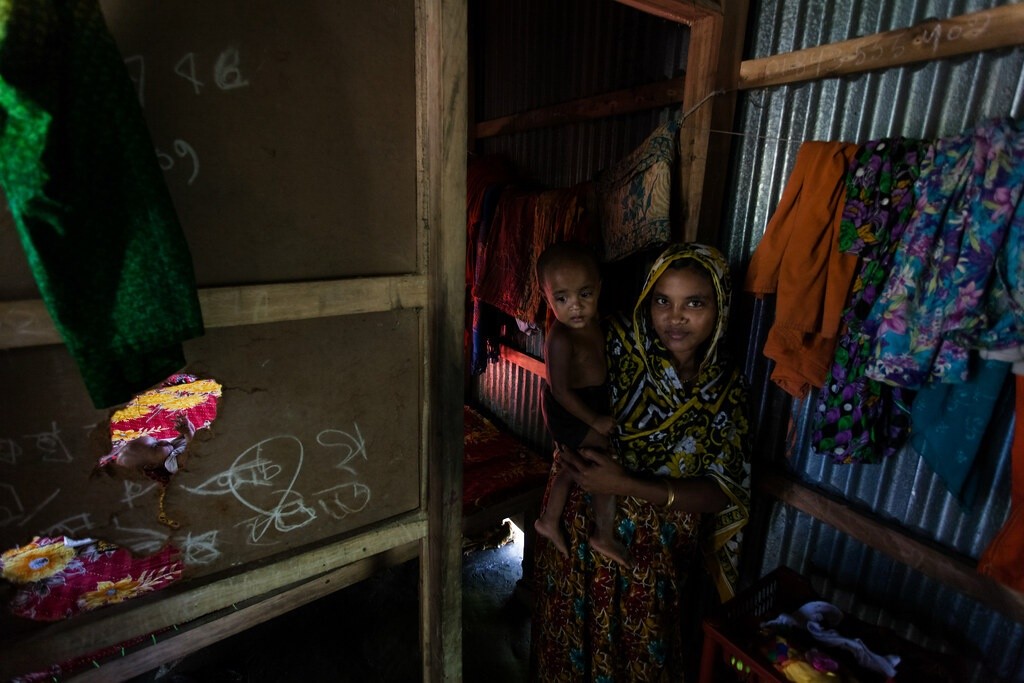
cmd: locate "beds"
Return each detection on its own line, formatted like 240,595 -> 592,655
464,340 -> 551,576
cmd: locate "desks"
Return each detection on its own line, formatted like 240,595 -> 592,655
699,614 -> 791,683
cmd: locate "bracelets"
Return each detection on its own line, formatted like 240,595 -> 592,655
652,478 -> 677,511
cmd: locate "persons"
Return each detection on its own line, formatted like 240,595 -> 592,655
534,243 -> 754,683
536,243 -> 634,571
